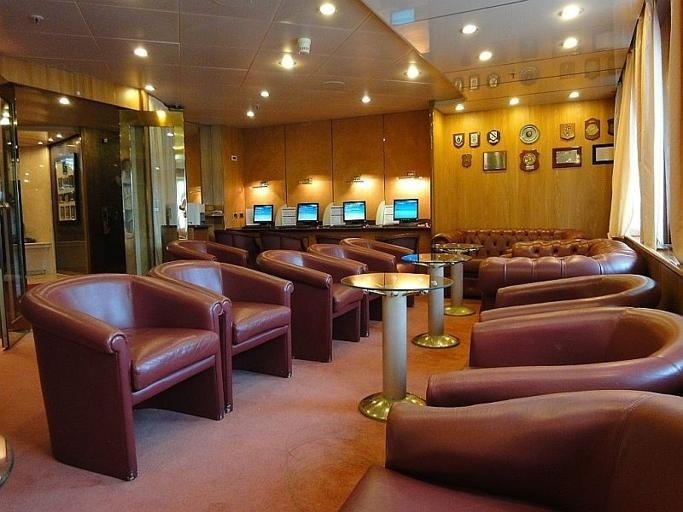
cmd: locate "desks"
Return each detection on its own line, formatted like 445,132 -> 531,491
228,225 -> 431,254
341,273 -> 455,422
399,253 -> 472,349
432,242 -> 483,317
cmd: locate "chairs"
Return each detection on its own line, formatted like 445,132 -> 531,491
258,231 -> 303,251
334,390 -> 683,512
477,274 -> 662,321
18,273 -> 224,480
146,260 -> 295,413
479,239 -> 645,317
308,244 -> 393,337
425,306 -> 683,408
255,250 -> 361,362
374,232 -> 419,251
341,237 -> 416,309
316,234 -> 359,244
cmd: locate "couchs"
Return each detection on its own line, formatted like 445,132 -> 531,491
213,229 -> 256,266
167,241 -> 248,267
432,228 -> 611,298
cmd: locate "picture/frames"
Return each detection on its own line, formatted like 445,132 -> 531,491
483,150 -> 507,171
552,146 -> 583,168
54,152 -> 78,222
591,143 -> 614,165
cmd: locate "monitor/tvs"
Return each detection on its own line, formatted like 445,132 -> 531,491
393,198 -> 419,221
252,204 -> 273,223
296,202 -> 319,223
343,200 -> 366,222
